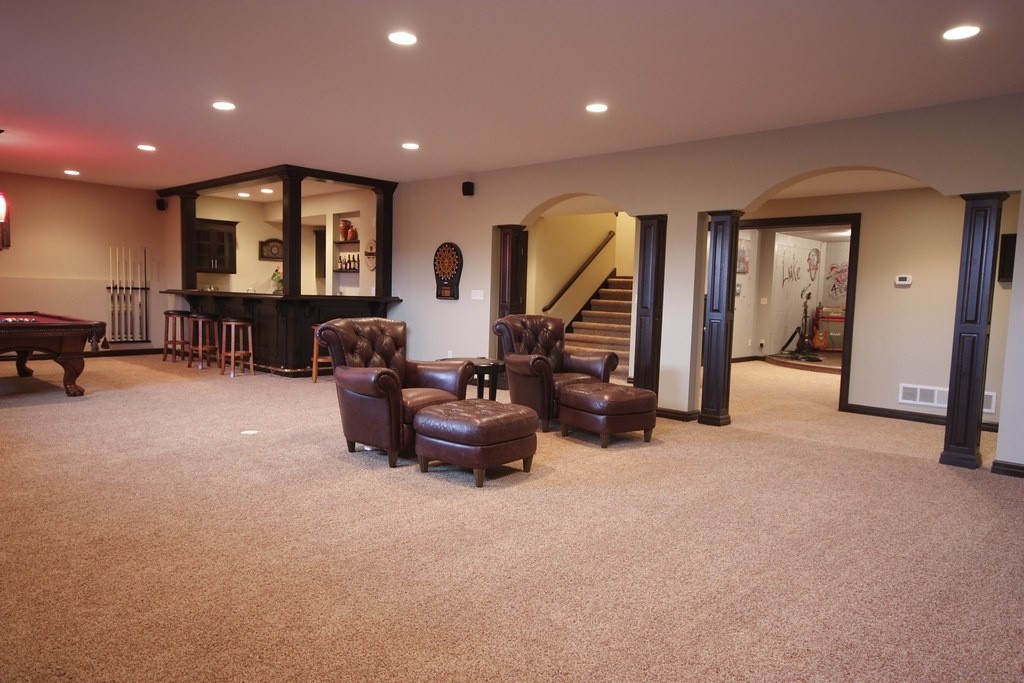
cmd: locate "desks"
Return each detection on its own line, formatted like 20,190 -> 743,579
0,310 -> 110,397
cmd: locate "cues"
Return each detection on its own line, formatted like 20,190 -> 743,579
108,244 -> 149,341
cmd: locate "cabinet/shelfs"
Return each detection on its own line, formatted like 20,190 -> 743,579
195,217 -> 238,274
333,240 -> 360,274
312,229 -> 326,278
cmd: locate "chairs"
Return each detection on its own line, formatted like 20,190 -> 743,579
317,315 -> 474,470
492,314 -> 619,435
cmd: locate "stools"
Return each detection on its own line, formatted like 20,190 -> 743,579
311,321 -> 332,383
412,398 -> 539,487
559,380 -> 658,449
220,318 -> 256,378
188,314 -> 220,370
162,309 -> 190,362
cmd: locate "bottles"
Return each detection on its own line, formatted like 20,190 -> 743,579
342,256 -> 347,269
355,254 -> 359,269
351,255 -> 355,269
346,255 -> 351,269
348,225 -> 355,240
338,256 -> 342,269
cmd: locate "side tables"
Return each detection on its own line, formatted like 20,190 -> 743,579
437,357 -> 506,402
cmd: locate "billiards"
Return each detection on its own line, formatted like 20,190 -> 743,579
1,316 -> 36,322
